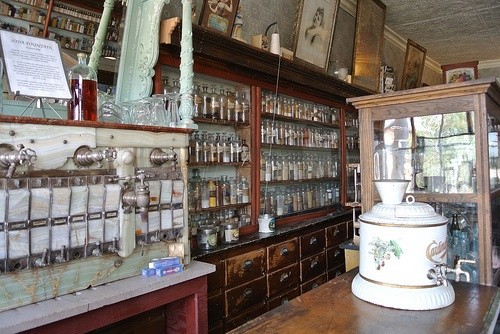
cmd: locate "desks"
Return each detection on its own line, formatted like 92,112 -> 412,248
225,267 -> 500,334
0,262 -> 218,334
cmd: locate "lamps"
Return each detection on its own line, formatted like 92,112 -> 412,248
262,20 -> 281,56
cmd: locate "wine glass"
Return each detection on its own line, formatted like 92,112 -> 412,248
120,93 -> 180,128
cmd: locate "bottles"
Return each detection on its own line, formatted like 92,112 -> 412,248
190,131 -> 249,162
160,75 -> 171,93
423,156 -> 479,277
194,82 -> 249,122
232,16 -> 243,38
345,114 -> 360,150
171,79 -> 180,93
3,3 -> 118,56
258,215 -> 276,234
188,169 -> 251,250
261,93 -> 340,215
67,53 -> 98,121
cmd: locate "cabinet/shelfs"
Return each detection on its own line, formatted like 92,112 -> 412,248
345,76 -> 500,286
153,18 -> 362,334
0,0 -> 126,85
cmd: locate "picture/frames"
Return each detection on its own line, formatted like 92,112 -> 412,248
400,38 -> 427,89
292,0 -> 341,73
198,0 -> 239,37
352,0 -> 387,92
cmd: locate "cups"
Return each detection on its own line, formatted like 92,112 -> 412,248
338,68 -> 349,81
373,179 -> 411,205
